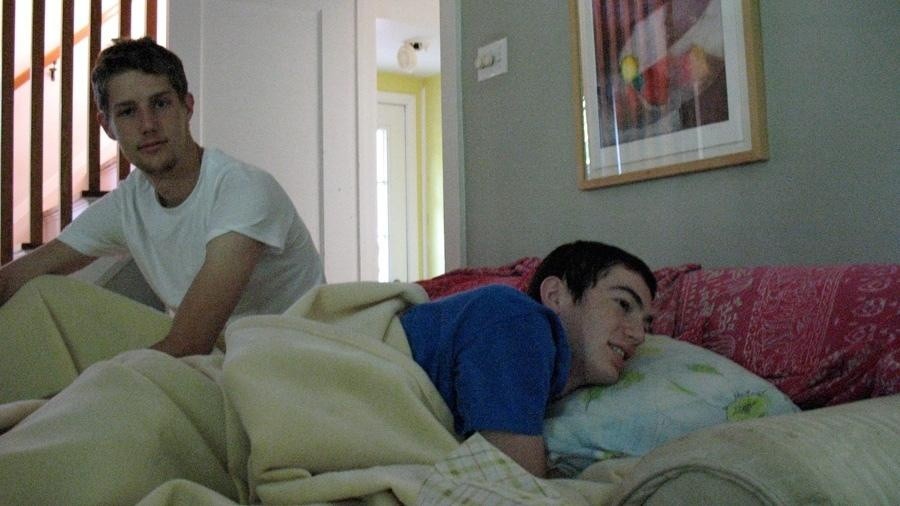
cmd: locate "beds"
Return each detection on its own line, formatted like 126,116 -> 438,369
0,254 -> 900,506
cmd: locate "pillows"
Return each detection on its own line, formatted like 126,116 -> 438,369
539,323 -> 802,473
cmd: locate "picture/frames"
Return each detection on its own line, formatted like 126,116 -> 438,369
568,0 -> 770,193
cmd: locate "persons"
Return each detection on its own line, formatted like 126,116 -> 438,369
3,37 -> 328,400
398,240 -> 659,480
